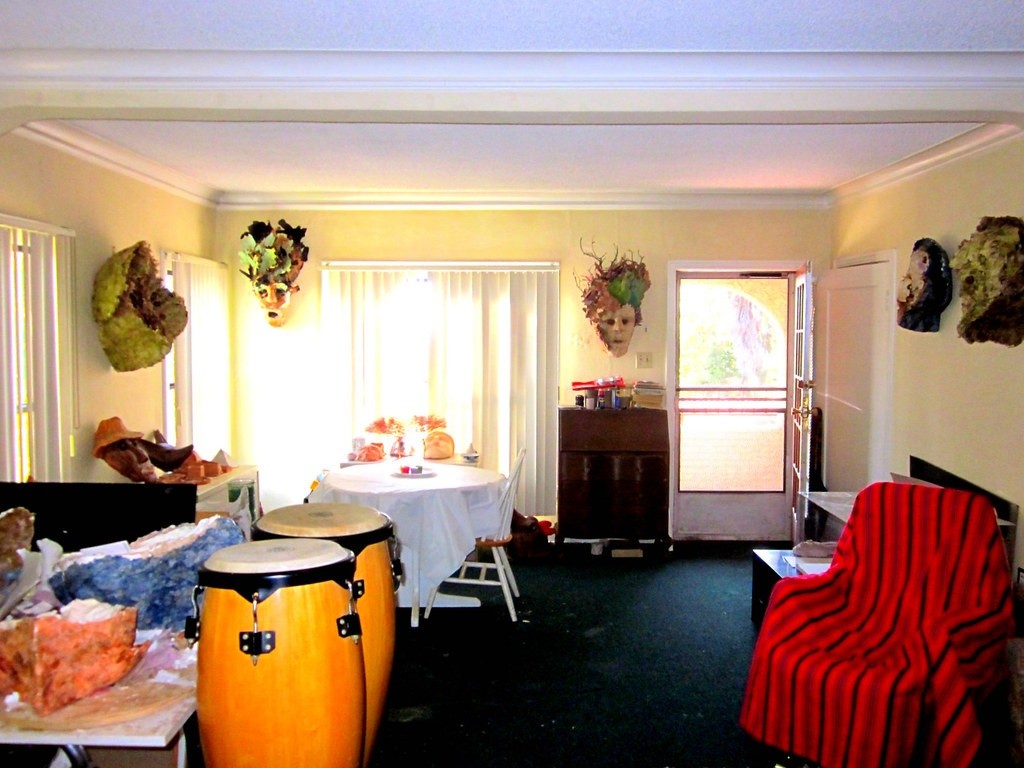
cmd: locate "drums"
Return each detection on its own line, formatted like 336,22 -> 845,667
194,537 -> 367,768
250,501 -> 398,768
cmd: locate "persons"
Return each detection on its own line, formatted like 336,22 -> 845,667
103,438 -> 158,483
598,303 -> 635,358
254,277 -> 291,327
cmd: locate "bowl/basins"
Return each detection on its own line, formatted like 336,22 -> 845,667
461,453 -> 479,462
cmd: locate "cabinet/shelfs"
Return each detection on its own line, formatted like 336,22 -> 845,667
138,464 -> 261,537
555,405 -> 672,546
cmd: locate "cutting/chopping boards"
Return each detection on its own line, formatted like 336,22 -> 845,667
0,661 -> 193,731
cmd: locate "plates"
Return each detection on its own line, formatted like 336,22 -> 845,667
396,468 -> 436,476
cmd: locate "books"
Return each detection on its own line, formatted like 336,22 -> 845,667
632,380 -> 666,408
608,540 -> 644,557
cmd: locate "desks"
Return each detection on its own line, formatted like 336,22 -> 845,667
307,456 -> 509,627
0,606 -> 215,747
751,548 -> 833,622
796,491 -> 1015,584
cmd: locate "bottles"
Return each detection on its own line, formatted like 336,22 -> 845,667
598,391 -> 605,409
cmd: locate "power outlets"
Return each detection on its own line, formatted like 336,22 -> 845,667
636,352 -> 651,368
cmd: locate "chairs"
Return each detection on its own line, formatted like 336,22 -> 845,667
424,447 -> 526,621
739,482 -> 1015,768
